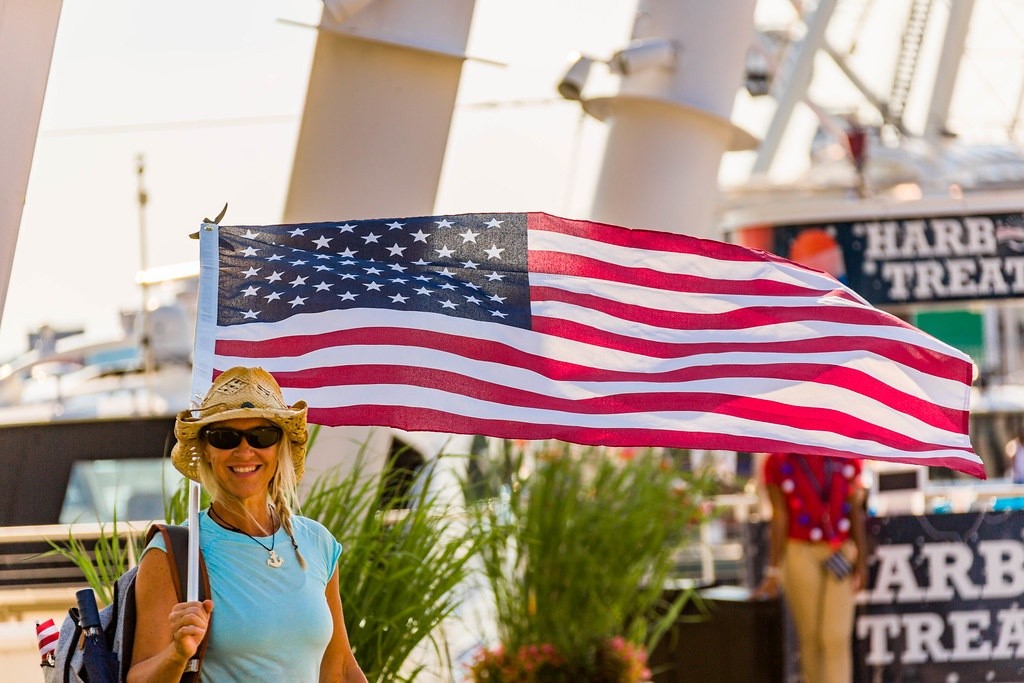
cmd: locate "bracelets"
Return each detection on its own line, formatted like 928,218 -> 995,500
761,566 -> 781,580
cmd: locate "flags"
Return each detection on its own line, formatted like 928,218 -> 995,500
33,619 -> 60,661
200,213 -> 987,477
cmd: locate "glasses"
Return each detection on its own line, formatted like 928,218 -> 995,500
198,426 -> 284,450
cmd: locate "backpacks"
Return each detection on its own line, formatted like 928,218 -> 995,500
36,525 -> 211,683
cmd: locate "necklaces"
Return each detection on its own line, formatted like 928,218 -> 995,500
209,502 -> 283,568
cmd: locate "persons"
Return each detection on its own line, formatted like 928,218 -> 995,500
122,366 -> 370,683
752,452 -> 869,683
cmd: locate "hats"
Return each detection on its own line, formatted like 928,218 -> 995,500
170,366 -> 308,484
